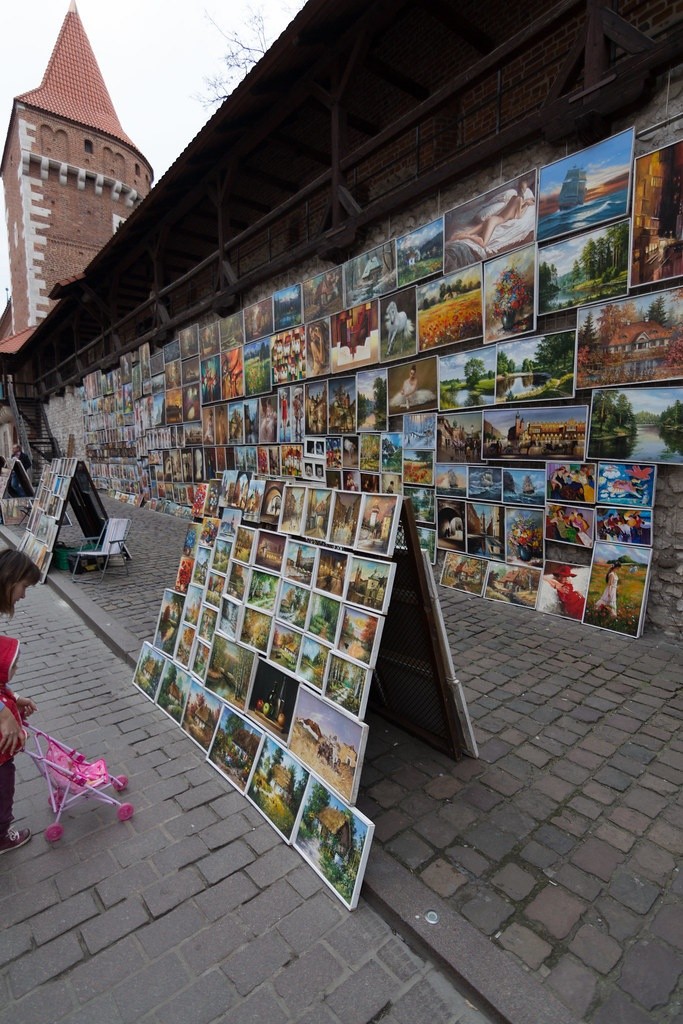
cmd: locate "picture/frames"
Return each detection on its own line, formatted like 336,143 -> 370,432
0,124 -> 683,915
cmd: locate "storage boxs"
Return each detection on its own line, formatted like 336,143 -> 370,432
53,543 -> 104,571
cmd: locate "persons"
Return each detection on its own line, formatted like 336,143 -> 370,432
280,387 -> 304,442
0,634 -> 31,854
596,560 -> 622,620
542,564 -> 585,620
548,464 -> 595,503
10,444 -> 32,474
204,405 -> 228,445
388,364 -> 436,410
0,547 -> 55,756
449,177 -> 537,249
260,397 -> 278,442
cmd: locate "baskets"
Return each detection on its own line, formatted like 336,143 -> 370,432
56,544 -> 103,569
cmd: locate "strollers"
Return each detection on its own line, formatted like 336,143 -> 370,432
16,720 -> 136,843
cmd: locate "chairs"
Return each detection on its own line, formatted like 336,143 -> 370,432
69,515 -> 134,587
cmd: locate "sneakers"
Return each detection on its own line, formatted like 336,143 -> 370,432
0,825 -> 31,854
